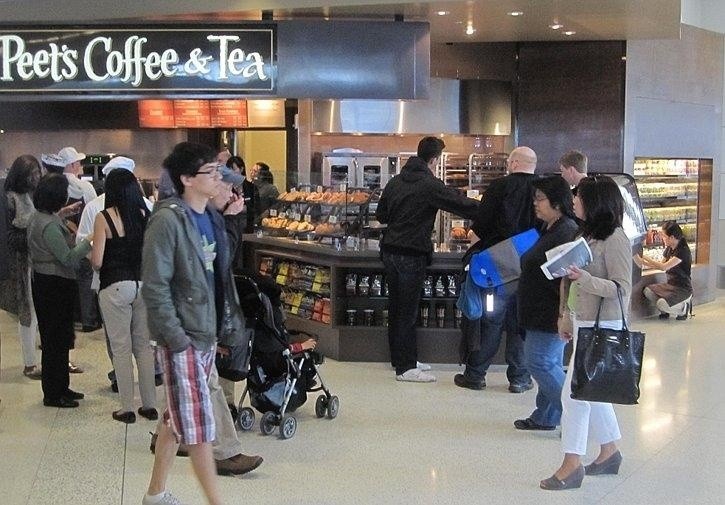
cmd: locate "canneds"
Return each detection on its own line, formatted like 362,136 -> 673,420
362,309 -> 374,327
419,301 -> 466,329
344,309 -> 358,326
381,306 -> 388,327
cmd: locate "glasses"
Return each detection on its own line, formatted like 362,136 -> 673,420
194,165 -> 222,178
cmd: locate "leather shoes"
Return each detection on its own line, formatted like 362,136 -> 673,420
676,302 -> 689,320
110,359 -> 164,420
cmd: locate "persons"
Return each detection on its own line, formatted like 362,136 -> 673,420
455,145 -> 545,394
513,176 -> 580,431
149,165 -> 263,475
141,140 -> 242,505
2,147 -> 163,423
538,175 -> 633,491
633,234 -> 651,310
639,221 -> 694,320
559,149 -> 589,195
157,145 -> 281,424
377,136 -> 482,383
290,338 -> 316,353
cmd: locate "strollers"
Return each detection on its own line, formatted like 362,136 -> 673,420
227,270 -> 339,438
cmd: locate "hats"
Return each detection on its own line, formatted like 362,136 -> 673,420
218,163 -> 246,186
41,147 -> 87,169
102,156 -> 135,177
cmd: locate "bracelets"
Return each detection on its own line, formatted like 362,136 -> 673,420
557,311 -> 564,319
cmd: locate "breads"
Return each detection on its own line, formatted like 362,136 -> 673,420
450,226 -> 467,240
260,217 -> 342,235
277,190 -> 370,204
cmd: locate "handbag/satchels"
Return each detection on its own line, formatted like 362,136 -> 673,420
570,326 -> 644,405
216,312 -> 256,381
470,227 -> 541,323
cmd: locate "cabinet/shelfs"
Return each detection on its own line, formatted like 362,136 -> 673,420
322,149 -> 511,249
253,172 -> 558,362
627,158 -> 713,276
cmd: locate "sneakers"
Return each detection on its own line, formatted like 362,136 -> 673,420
38,341 -> 77,351
396,366 -> 435,382
149,431 -> 193,455
217,452 -> 263,477
45,385 -> 84,407
83,322 -> 102,330
456,372 -> 484,390
515,416 -> 557,431
68,362 -> 83,376
144,493 -> 182,505
416,358 -> 432,370
23,364 -> 42,376
510,381 -> 534,392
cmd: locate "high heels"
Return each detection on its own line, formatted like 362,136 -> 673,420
585,451 -> 622,477
539,465 -> 585,489
